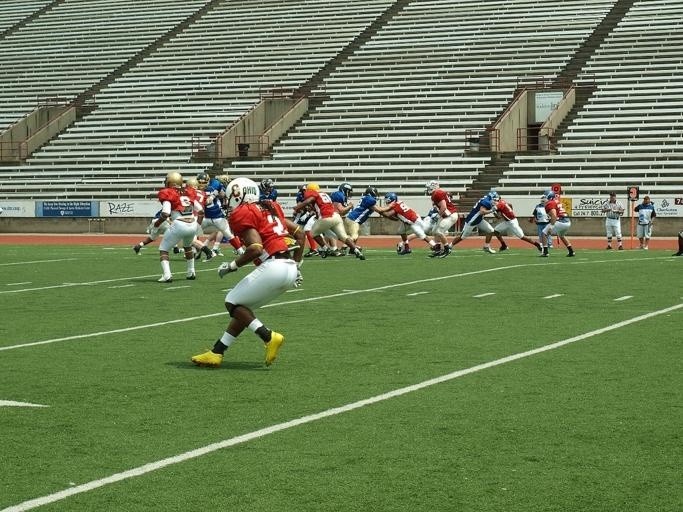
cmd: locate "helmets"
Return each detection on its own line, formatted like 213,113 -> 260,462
306,182 -> 319,193
339,183 -> 352,197
260,178 -> 276,195
185,179 -> 198,189
365,186 -> 377,197
299,184 -> 308,192
166,173 -> 183,187
197,175 -> 232,187
543,190 -> 556,200
491,197 -> 501,206
385,192 -> 397,204
225,178 -> 260,208
424,180 -> 440,194
487,191 -> 499,201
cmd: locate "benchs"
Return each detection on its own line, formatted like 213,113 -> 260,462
0,0 -> 212,136
1,1 -> 53,35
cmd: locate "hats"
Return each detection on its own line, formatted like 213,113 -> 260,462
644,196 -> 649,199
610,192 -> 615,196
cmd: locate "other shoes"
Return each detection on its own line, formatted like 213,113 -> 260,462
134,245 -> 142,256
157,274 -> 173,281
397,242 -> 412,255
566,252 -> 575,257
536,243 -> 544,252
427,243 -> 452,259
174,246 -> 224,263
237,247 -> 244,255
539,253 -> 550,257
483,247 -> 496,254
498,244 -> 508,251
186,272 -> 195,279
304,246 -> 366,260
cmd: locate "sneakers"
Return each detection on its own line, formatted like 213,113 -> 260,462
606,245 -> 612,249
191,350 -> 223,368
672,252 -> 683,256
619,245 -> 623,249
264,331 -> 285,368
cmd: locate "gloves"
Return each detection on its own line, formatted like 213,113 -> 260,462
293,262 -> 303,282
212,190 -> 219,196
217,261 -> 238,279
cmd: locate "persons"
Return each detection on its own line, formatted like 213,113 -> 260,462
602,191 -> 626,250
672,231 -> 683,256
635,196 -> 656,250
191,177 -> 306,367
133,169 -> 279,283
292,180 -> 576,260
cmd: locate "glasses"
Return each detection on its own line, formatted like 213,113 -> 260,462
198,181 -> 208,184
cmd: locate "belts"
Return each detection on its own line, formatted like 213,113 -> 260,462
266,254 -> 292,259
